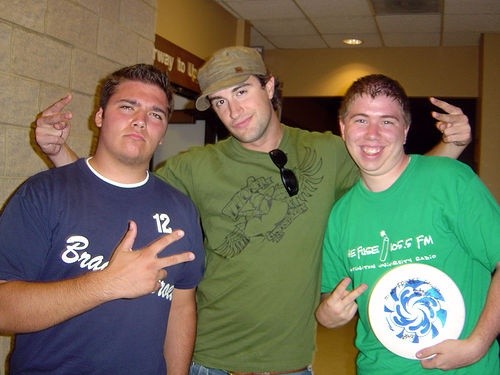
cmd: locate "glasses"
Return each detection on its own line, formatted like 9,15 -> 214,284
269,149 -> 299,196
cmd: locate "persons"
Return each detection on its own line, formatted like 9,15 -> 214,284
34,46 -> 472,374
1,65 -> 205,375
313,74 -> 500,374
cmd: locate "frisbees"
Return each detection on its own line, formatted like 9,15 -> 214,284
367,263 -> 466,360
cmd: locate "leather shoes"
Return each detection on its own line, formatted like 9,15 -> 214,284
226,367 -> 308,375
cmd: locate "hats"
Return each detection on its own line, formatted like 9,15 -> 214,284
196,45 -> 268,112
